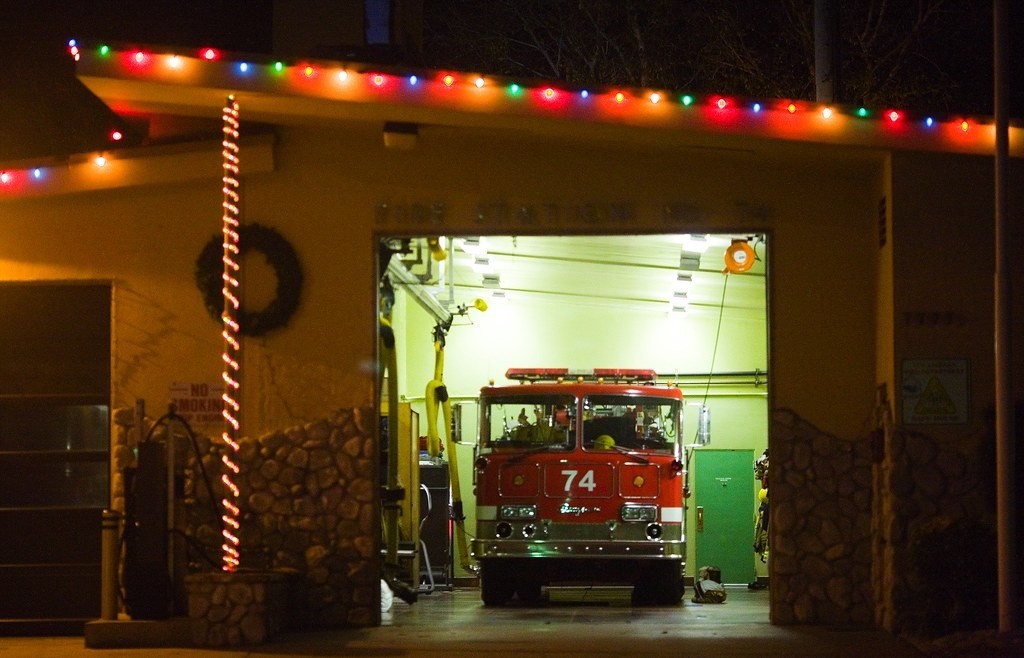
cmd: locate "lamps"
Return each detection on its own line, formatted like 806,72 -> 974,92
382,122 -> 419,152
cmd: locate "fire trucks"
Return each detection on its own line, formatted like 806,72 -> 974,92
449,366 -> 713,603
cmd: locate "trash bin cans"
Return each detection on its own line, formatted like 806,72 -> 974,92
418,462 -> 450,586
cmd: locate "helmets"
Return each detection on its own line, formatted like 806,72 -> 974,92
758,488 -> 769,504
594,435 -> 615,450
